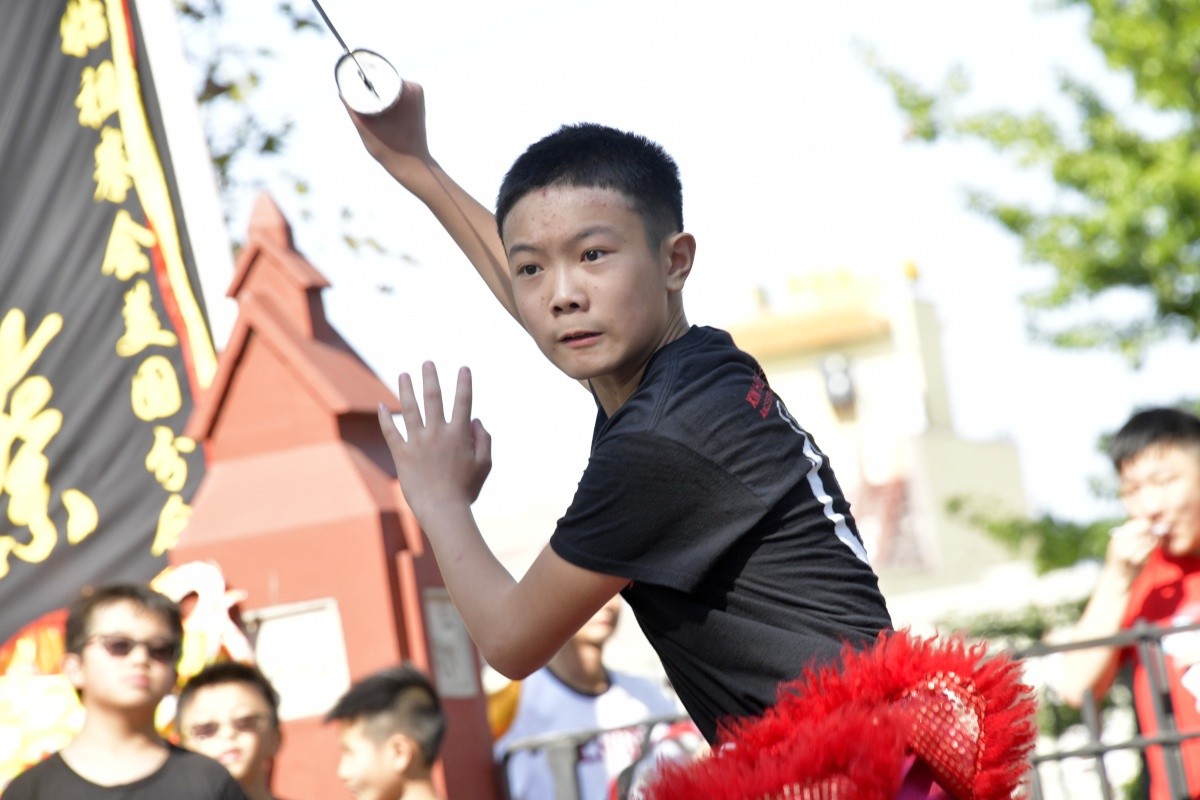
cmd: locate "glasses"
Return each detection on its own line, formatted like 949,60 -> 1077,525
72,634 -> 176,663
185,715 -> 261,744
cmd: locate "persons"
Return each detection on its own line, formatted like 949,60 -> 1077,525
485,591 -> 711,800
323,664 -> 448,800
1054,408 -> 1200,800
335,79 -> 896,761
175,660 -> 291,800
0,582 -> 251,800
